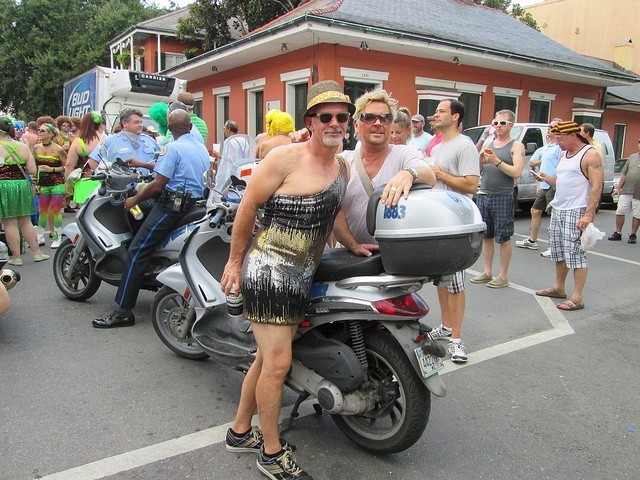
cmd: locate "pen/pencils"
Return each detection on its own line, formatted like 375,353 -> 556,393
462,124 -> 615,220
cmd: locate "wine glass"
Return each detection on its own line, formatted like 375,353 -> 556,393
391,187 -> 397,192
125,205 -> 127,209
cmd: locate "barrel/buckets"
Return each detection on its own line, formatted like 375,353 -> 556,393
556,297 -> 585,311
536,287 -> 568,299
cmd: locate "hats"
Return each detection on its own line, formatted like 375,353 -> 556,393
603,158 -> 630,211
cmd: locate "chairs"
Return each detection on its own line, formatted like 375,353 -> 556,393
256,442 -> 314,480
514,238 -> 538,250
627,234 -> 637,243
469,273 -> 492,284
608,231 -> 621,240
426,324 -> 452,340
540,247 -> 552,258
7,258 -> 22,266
486,276 -> 508,289
226,426 -> 296,455
33,254 -> 50,262
447,339 -> 467,363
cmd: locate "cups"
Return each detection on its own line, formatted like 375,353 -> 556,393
62,124 -> 70,126
548,125 -> 554,128
37,127 -> 44,132
307,112 -> 351,123
360,112 -> 393,125
493,119 -> 512,126
168,101 -> 172,111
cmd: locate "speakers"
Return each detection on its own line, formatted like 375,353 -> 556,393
37,225 -> 45,245
51,226 -> 62,248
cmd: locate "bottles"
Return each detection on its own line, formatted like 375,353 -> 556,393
301,80 -> 356,125
427,113 -> 436,120
551,120 -> 581,136
145,125 -> 157,134
412,114 -> 425,121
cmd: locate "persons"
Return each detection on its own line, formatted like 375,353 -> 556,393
470,110 -> 525,287
55,116 -> 76,213
259,113 -> 295,158
255,109 -> 287,158
1,116 -> 49,265
165,102 -> 204,146
208,120 -> 238,159
14,121 -> 39,226
289,88 -> 437,246
1,281 -> 10,316
99,116 -> 108,141
36,116 -> 56,126
65,111 -> 107,210
430,99 -> 480,201
516,118 -> 567,257
178,94 -> 208,144
32,123 -> 66,249
72,117 -> 82,136
221,80 -> 380,480
389,107 -> 412,144
23,121 -> 38,147
426,114 -> 444,157
608,139 -> 640,244
88,108 -> 161,209
580,122 -> 604,161
92,109 -> 210,327
536,121 -> 604,311
407,113 -> 432,151
147,102 -> 168,135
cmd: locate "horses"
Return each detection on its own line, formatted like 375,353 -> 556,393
530,170 -> 543,180
485,149 -> 492,154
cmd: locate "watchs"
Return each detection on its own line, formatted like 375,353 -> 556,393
53,145 -> 210,302
150,169 -> 488,452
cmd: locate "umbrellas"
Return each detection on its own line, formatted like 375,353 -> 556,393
91,310 -> 135,328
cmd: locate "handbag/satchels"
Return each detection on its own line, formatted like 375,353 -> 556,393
496,160 -> 502,168
402,167 -> 417,183
